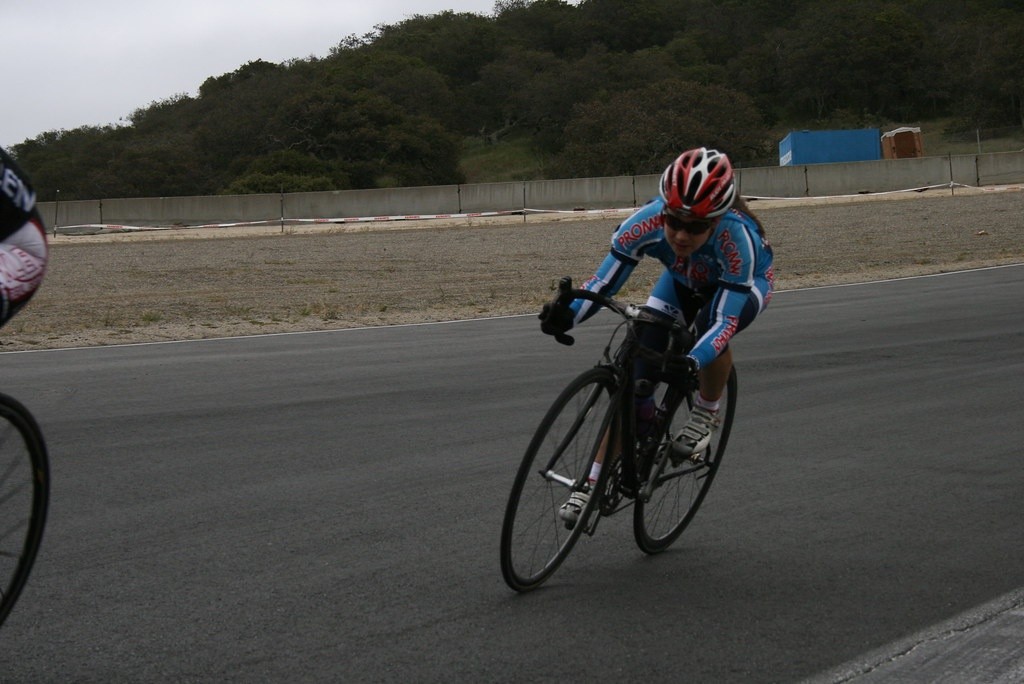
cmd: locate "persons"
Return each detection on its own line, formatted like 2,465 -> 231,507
0,147 -> 49,329
540,148 -> 772,524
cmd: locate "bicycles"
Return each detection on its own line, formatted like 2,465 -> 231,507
498,274 -> 740,595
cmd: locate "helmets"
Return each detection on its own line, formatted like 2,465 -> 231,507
658,147 -> 737,218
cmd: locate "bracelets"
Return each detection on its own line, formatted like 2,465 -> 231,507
686,354 -> 699,371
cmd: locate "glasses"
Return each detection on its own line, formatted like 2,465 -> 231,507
662,206 -> 713,234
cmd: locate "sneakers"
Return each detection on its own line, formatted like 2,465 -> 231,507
672,405 -> 721,454
558,481 -> 597,521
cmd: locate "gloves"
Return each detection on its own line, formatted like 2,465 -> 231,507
660,350 -> 691,386
537,303 -> 574,335
